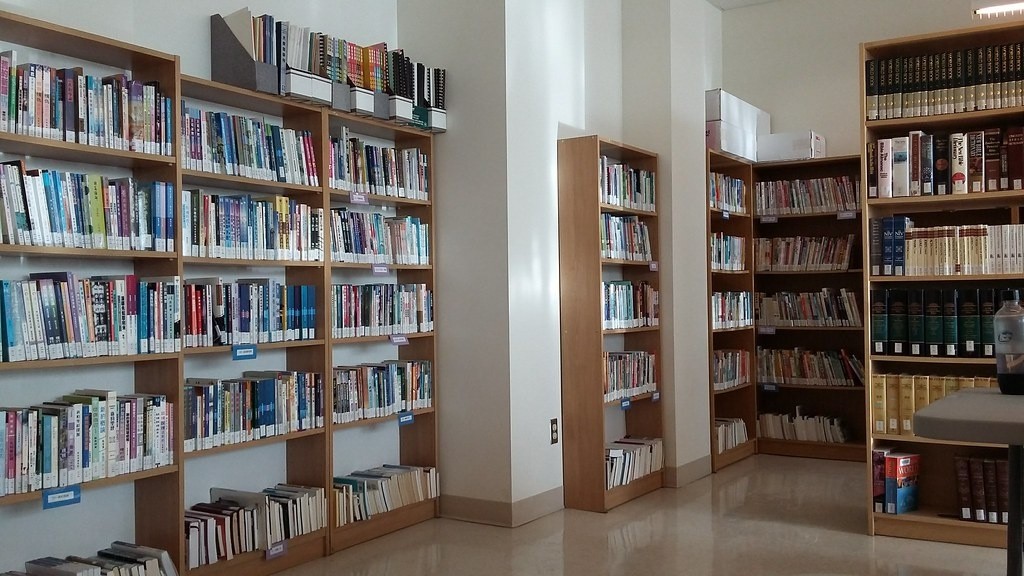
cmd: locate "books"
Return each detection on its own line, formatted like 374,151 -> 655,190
599,43 -> 1023,525
2,12 -> 448,576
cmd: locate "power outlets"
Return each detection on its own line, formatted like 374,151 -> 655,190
550,418 -> 558,445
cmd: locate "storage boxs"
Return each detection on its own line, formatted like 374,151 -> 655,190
706,88 -> 771,135
757,130 -> 826,162
705,121 -> 756,164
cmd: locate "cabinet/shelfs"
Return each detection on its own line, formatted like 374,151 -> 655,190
860,20 -> 1024,550
706,148 -> 758,472
180,72 -> 329,576
0,10 -> 184,576
327,108 -> 441,555
559,135 -> 668,512
755,155 -> 868,463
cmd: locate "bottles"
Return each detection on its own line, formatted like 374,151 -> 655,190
994,289 -> 1024,395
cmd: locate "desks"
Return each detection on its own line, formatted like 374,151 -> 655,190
913,385 -> 1024,576
